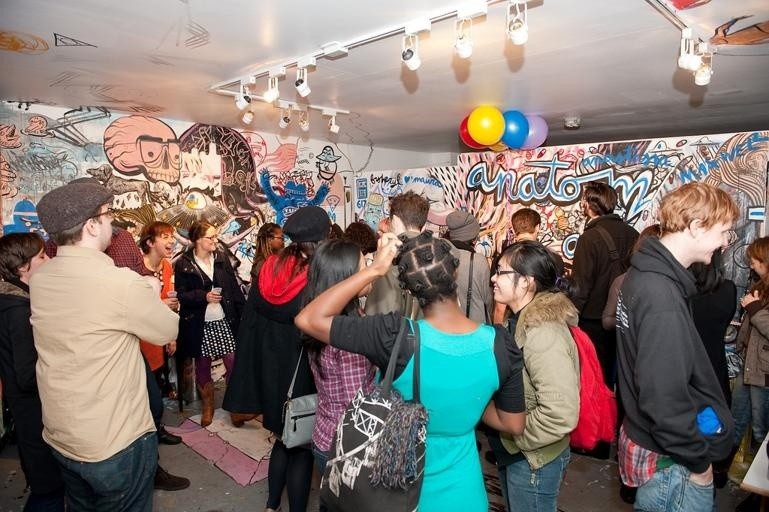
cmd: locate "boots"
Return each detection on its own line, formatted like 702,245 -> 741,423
197,380 -> 215,428
231,413 -> 256,427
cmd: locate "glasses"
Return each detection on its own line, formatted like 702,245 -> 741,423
495,268 -> 516,275
204,236 -> 214,240
93,208 -> 115,218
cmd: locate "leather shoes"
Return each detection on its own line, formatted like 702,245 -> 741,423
154,464 -> 190,491
156,425 -> 181,445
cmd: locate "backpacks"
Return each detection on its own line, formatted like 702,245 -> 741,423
567,323 -> 619,450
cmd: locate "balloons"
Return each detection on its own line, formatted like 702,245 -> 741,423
467,105 -> 505,145
490,142 -> 507,151
520,115 -> 548,151
459,116 -> 489,150
501,111 -> 528,149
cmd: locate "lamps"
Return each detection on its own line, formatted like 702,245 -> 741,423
207,0 -> 719,134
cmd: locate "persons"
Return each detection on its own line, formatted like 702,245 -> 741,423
1,179 -> 769,511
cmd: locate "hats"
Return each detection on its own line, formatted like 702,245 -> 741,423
37,183 -> 113,234
282,206 -> 329,242
446,211 -> 480,241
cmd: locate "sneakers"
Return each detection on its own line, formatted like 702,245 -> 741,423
570,448 -> 609,461
485,451 -> 497,464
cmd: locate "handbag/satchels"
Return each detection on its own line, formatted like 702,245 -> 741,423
320,383 -> 426,511
282,393 -> 318,448
582,262 -> 626,319
